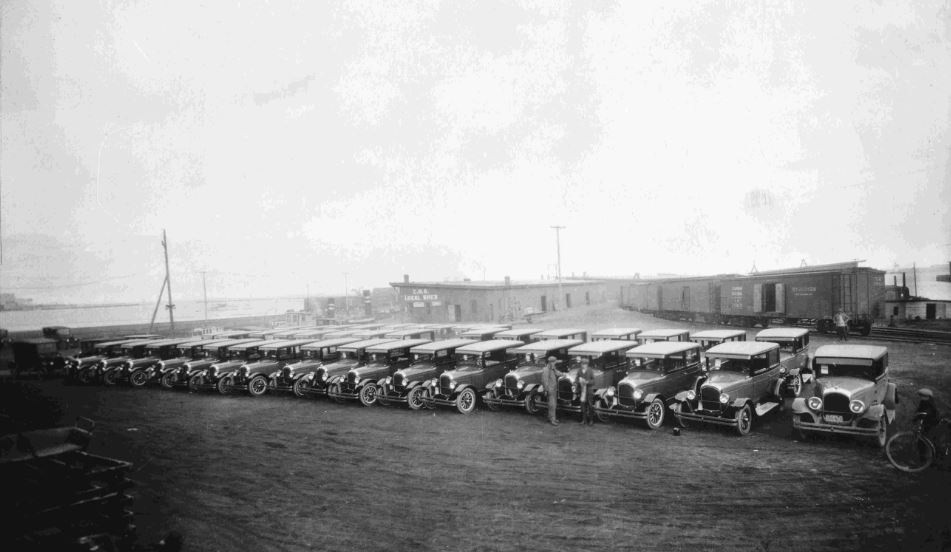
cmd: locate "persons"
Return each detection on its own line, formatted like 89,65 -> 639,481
911,388 -> 941,464
574,358 -> 595,427
832,308 -> 849,342
541,355 -> 568,426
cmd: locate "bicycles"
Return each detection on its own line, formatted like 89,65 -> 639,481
885,412 -> 951,473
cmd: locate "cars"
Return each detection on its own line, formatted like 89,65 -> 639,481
670,341 -> 791,437
49,323 -> 690,422
43,326 -> 80,350
0,328 -> 13,349
691,330 -> 747,372
754,328 -> 815,396
10,337 -> 60,380
593,340 -> 707,428
789,343 -> 901,446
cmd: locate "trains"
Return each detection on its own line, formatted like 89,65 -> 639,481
616,266 -> 886,338
